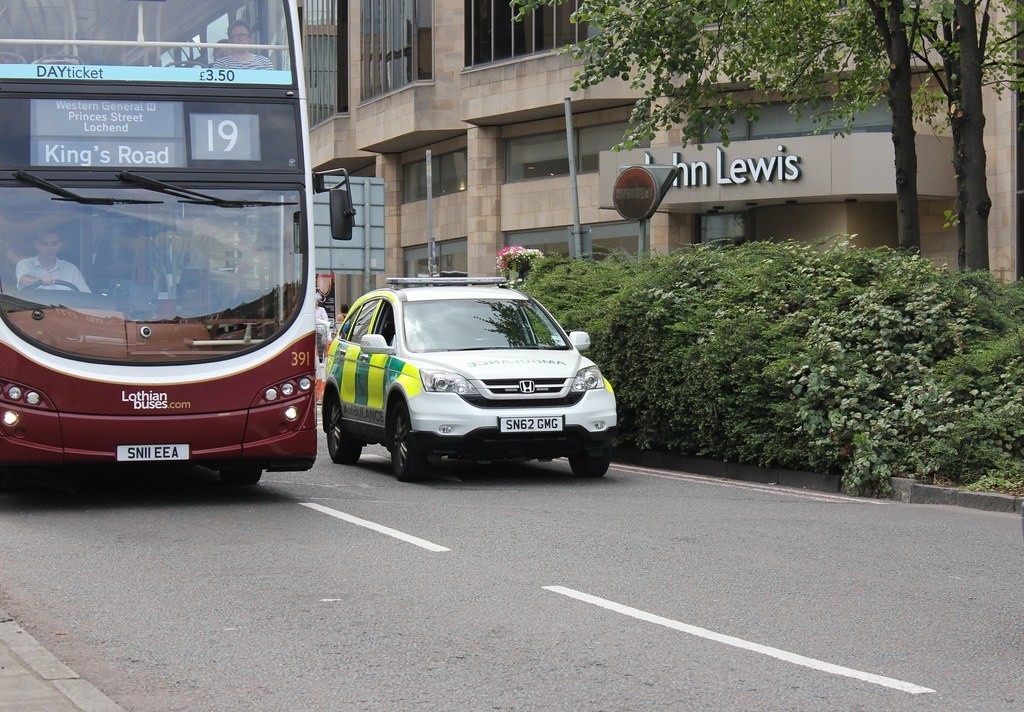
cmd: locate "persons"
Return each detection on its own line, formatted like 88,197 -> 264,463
13,227 -> 93,295
204,20 -> 275,70
315,291 -> 332,341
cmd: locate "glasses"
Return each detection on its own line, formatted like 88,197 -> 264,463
38,239 -> 60,247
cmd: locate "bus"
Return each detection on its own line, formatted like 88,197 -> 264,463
0,0 -> 356,493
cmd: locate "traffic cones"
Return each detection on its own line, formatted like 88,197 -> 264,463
314,343 -> 331,406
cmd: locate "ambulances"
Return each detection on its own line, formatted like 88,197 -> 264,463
321,275 -> 617,482
